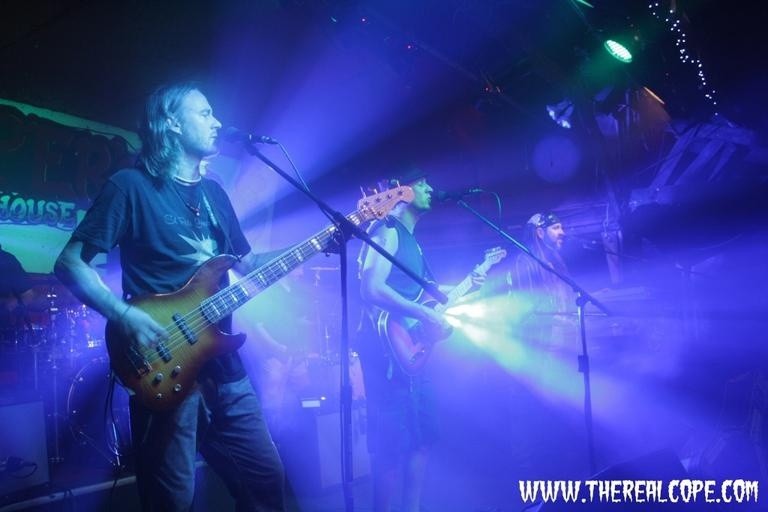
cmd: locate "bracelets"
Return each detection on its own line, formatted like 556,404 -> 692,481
116,300 -> 133,326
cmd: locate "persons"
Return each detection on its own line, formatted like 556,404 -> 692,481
1,245 -> 34,334
513,209 -> 610,364
351,161 -> 489,512
52,80 -> 365,510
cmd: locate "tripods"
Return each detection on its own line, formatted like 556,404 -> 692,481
44,282 -> 116,468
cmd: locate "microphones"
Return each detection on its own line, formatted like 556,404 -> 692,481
224,126 -> 279,144
434,188 -> 483,202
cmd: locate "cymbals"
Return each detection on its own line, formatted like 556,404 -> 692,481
18,277 -> 63,287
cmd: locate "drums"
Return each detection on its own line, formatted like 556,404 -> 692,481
64,354 -> 133,465
56,302 -> 106,350
0,327 -> 47,367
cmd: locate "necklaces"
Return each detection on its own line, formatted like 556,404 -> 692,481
170,179 -> 203,217
172,174 -> 206,186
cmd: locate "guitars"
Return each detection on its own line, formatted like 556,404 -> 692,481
377,247 -> 507,376
105,180 -> 414,409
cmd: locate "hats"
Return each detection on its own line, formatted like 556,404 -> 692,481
388,168 -> 428,190
526,212 -> 561,228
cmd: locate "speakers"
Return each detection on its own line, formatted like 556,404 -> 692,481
534,449 -> 696,511
